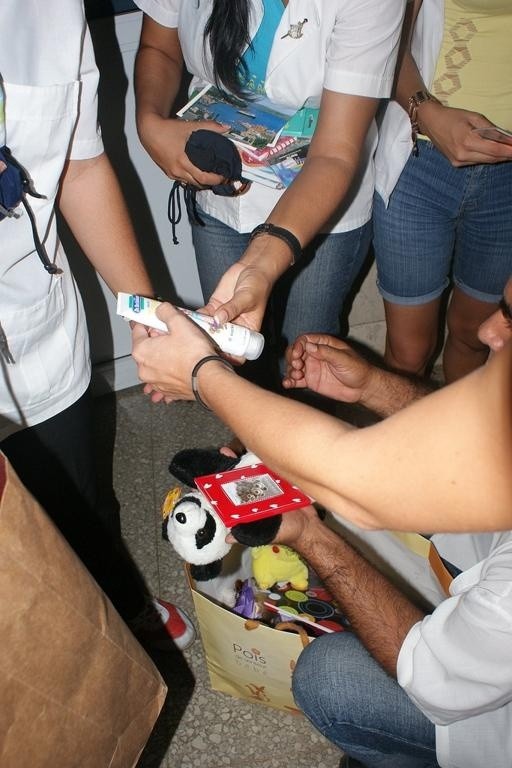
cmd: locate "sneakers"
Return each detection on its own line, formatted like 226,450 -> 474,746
128,597 -> 192,649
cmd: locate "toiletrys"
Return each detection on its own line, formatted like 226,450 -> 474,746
116,291 -> 265,361
